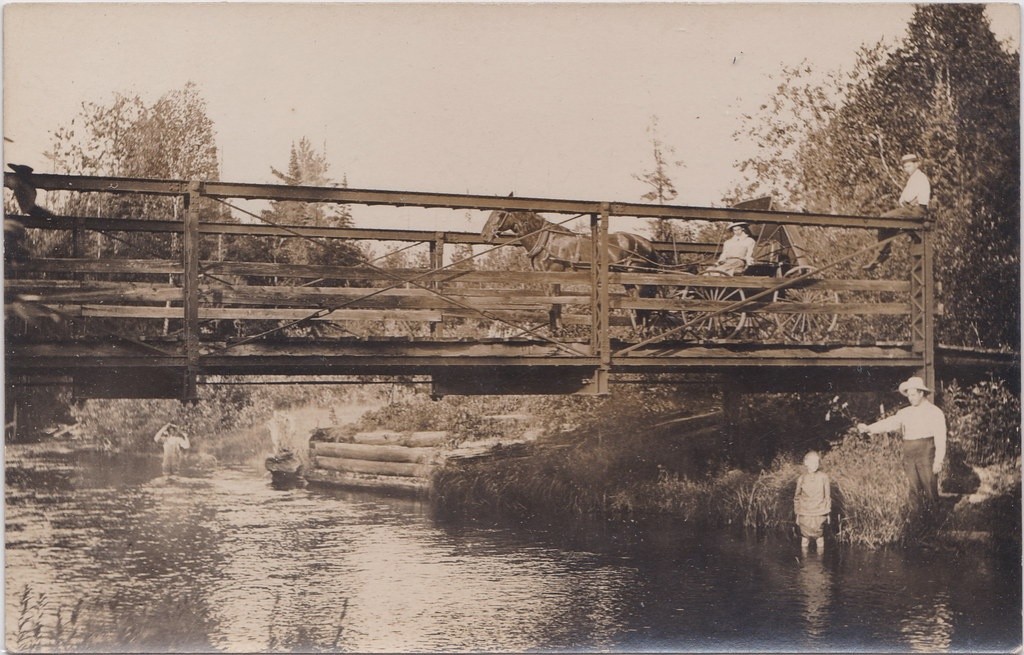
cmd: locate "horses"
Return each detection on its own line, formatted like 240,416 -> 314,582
481,192 -> 658,343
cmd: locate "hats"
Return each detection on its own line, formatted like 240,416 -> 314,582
898,154 -> 921,164
899,377 -> 932,396
726,221 -> 748,230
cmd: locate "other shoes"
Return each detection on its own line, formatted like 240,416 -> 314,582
863,263 -> 882,271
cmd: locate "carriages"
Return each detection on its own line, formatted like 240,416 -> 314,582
481,190 -> 839,342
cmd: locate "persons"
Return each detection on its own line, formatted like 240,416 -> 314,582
794,450 -> 834,551
862,155 -> 930,270
703,223 -> 757,278
153,423 -> 190,481
858,377 -> 946,523
